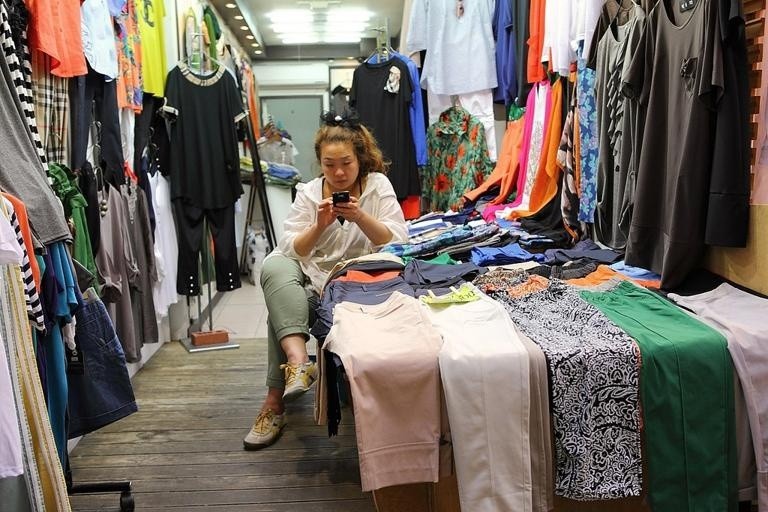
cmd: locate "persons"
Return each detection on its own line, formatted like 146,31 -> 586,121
244,105 -> 408,451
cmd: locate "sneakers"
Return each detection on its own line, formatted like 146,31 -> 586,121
281,361 -> 319,400
243,409 -> 288,449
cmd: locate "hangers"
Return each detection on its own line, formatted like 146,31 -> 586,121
359,28 -> 396,65
168,34 -> 225,80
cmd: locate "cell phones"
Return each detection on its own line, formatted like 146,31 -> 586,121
333,191 -> 350,208
